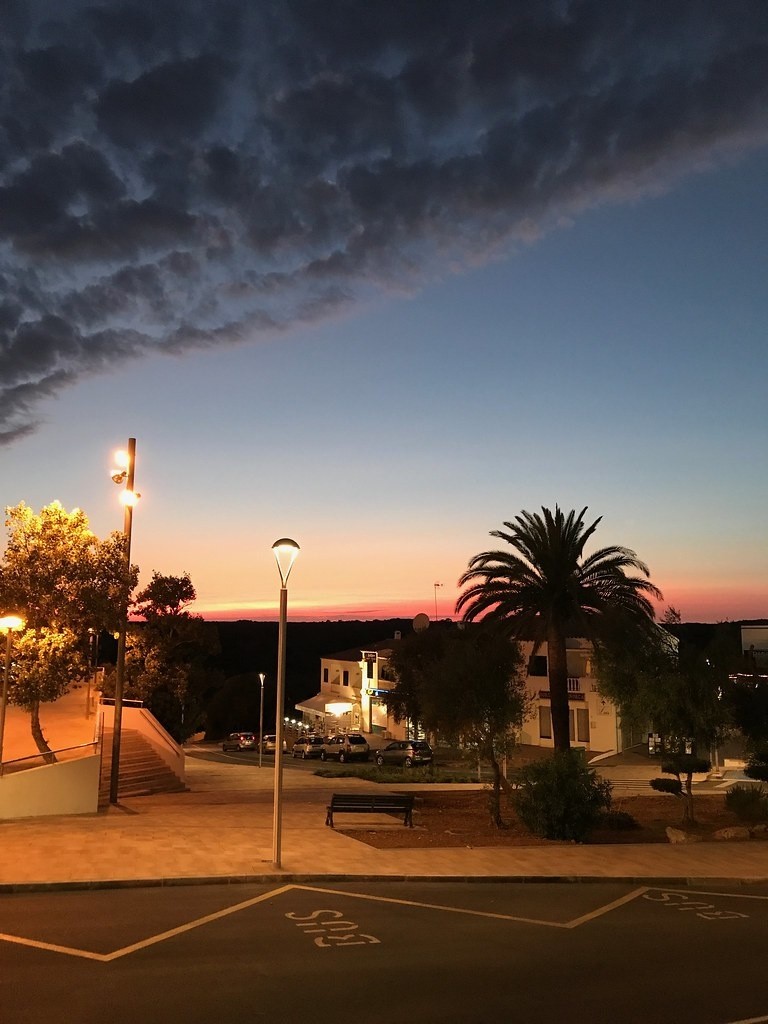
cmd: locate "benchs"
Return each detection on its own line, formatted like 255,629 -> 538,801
325,793 -> 414,827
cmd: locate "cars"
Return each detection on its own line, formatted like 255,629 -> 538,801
254,734 -> 287,754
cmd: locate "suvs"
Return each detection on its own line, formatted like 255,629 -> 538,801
291,734 -> 324,758
320,734 -> 369,763
374,739 -> 434,768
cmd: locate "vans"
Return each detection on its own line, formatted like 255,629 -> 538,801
222,731 -> 255,753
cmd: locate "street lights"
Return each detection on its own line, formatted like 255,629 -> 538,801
268,536 -> 302,876
102,401 -> 147,817
258,673 -> 265,769
434,582 -> 444,623
0,607 -> 28,781
84,627 -> 94,719
